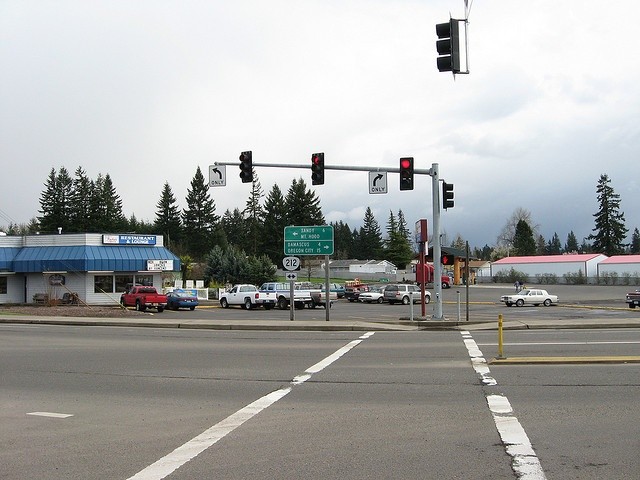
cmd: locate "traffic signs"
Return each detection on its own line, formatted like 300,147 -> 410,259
283,226 -> 334,256
209,165 -> 226,187
369,171 -> 387,194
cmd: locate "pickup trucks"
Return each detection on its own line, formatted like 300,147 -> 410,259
219,285 -> 277,310
120,285 -> 168,313
293,282 -> 337,308
258,282 -> 311,310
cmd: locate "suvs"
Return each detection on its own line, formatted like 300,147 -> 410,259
384,284 -> 431,305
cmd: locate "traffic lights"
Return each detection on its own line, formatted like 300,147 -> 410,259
310,152 -> 324,185
443,182 -> 454,209
399,157 -> 414,191
239,151 -> 252,183
435,18 -> 460,74
442,254 -> 455,265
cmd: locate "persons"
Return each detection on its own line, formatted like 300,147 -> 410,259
514,280 -> 519,293
519,279 -> 524,291
523,285 -> 526,289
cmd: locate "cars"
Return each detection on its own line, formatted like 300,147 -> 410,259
625,286 -> 640,307
164,291 -> 198,310
358,286 -> 395,304
500,289 -> 559,307
314,283 -> 347,299
